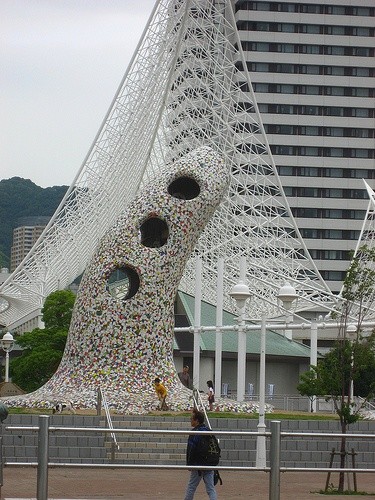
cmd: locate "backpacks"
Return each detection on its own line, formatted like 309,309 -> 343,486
195,427 -> 222,465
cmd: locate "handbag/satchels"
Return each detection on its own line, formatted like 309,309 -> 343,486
203,470 -> 223,487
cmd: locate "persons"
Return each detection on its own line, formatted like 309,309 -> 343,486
184,410 -> 217,500
154,378 -> 171,412
178,365 -> 191,390
207,381 -> 215,411
